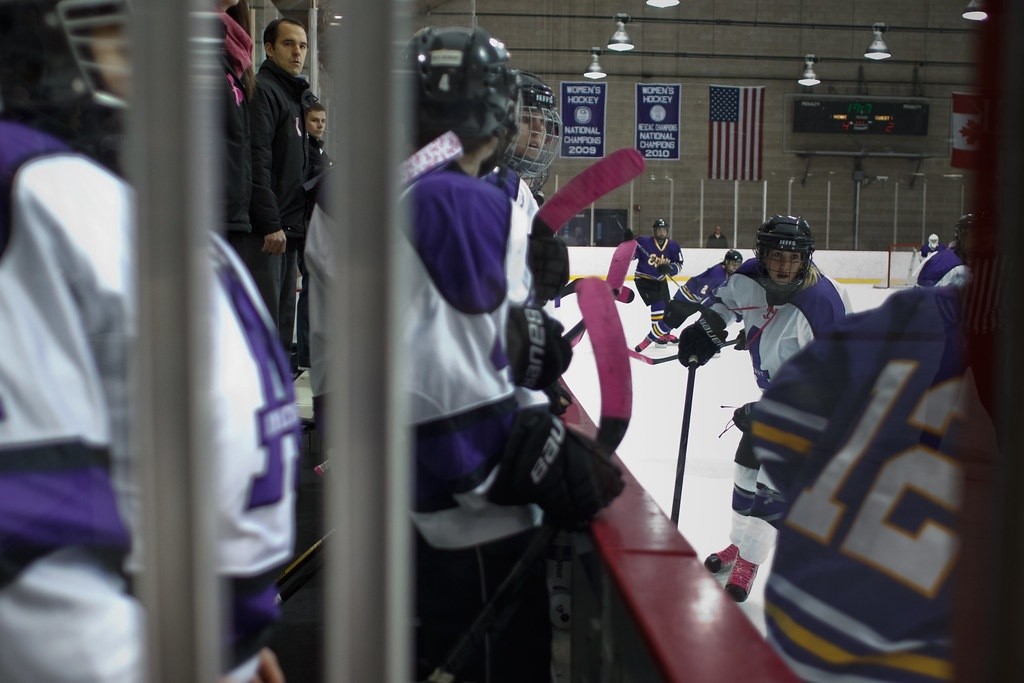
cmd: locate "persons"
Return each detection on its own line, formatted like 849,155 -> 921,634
749,207 -> 1001,683
634,250 -> 744,354
678,213 -> 845,606
0,0 -> 624,683
706,225 -> 729,248
624,219 -> 683,347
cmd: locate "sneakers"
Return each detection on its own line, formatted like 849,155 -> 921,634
705,543 -> 740,575
724,550 -> 758,603
635,338 -> 650,352
668,333 -> 679,345
655,335 -> 668,348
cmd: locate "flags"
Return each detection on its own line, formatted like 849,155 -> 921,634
950,91 -> 993,169
707,86 -> 763,182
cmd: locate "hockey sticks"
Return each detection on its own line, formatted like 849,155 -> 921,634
313,146 -> 649,480
558,276 -> 636,305
628,338 -> 740,365
670,354 -> 701,527
913,246 -> 924,261
270,238 -> 641,612
615,217 -> 681,288
429,273 -> 637,683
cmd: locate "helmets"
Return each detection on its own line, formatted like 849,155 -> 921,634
479,67 -> 561,192
755,214 -> 812,294
654,218 -> 669,240
723,249 -> 742,275
407,23 -> 517,176
954,214 -> 976,243
1,1 -> 133,171
928,234 -> 938,248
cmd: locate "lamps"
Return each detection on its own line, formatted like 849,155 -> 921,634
863,22 -> 892,59
583,47 -> 607,79
798,54 -> 821,86
606,13 -> 635,51
961,0 -> 988,20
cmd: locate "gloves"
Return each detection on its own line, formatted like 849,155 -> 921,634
484,407 -> 626,529
678,310 -> 728,368
524,233 -> 570,297
624,229 -> 633,242
507,305 -> 573,389
734,401 -> 758,431
656,263 -> 676,276
